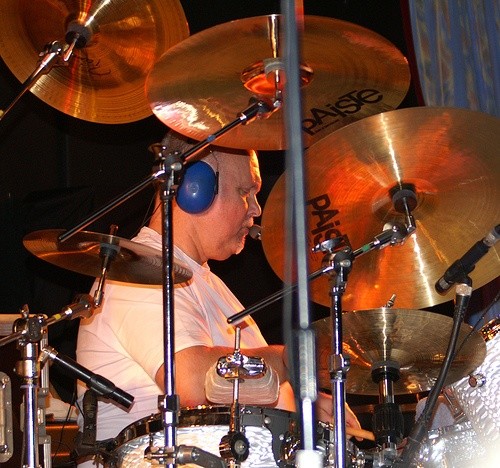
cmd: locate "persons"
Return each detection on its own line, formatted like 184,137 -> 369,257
75,128 -> 362,468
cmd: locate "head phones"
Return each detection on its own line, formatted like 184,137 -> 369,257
150,143 -> 217,215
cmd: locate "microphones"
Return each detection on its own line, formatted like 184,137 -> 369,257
248,224 -> 262,239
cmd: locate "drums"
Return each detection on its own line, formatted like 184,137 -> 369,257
449,312 -> 500,468
107,404 -> 366,468
414,421 -> 489,468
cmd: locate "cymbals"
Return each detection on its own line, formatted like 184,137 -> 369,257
0,0 -> 190,123
261,107 -> 500,311
282,309 -> 487,396
22,230 -> 194,286
146,16 -> 411,151
